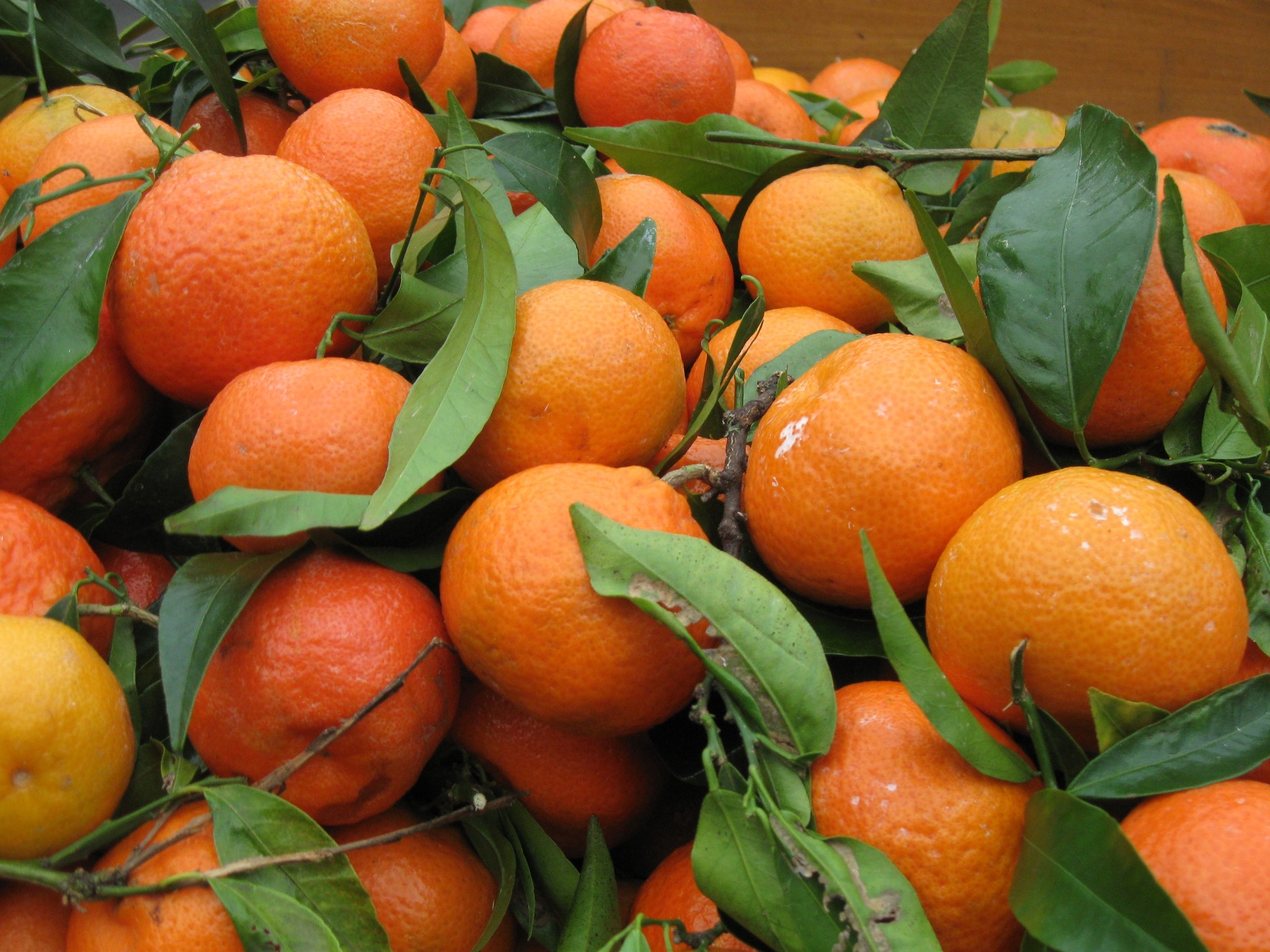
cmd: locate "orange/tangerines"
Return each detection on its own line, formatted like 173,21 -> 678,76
1,0 -> 1267,949
